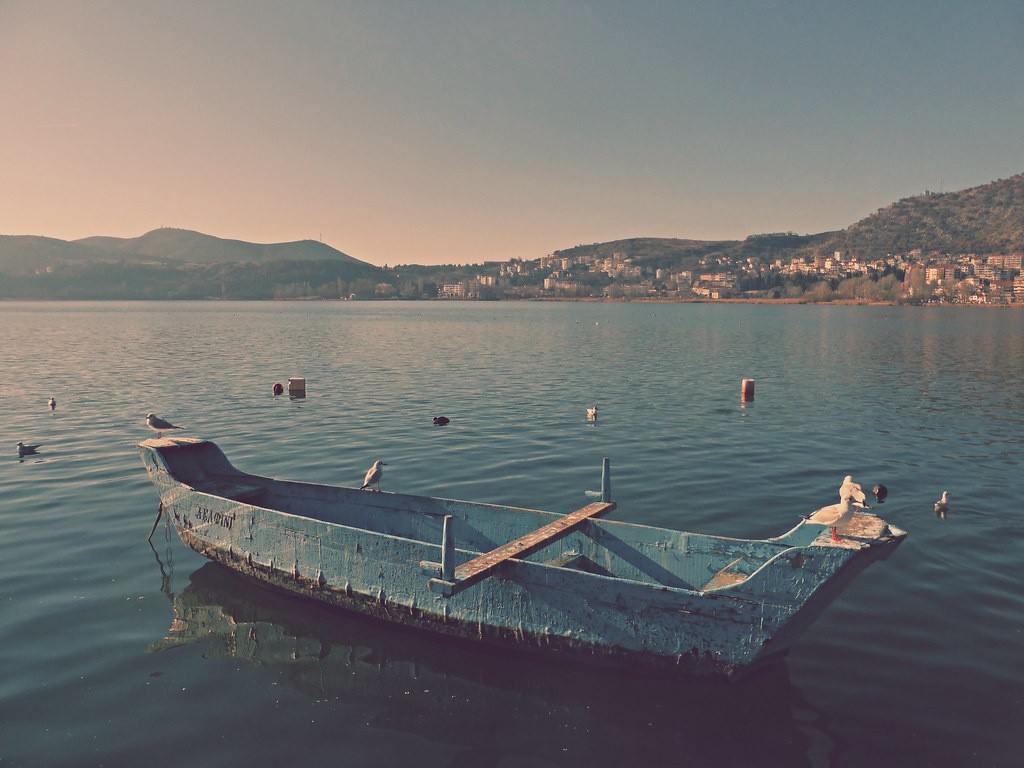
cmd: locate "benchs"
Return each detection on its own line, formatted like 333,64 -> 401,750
187,479 -> 266,502
698,557 -> 765,592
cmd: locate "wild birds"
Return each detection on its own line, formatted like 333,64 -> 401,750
432,416 -> 450,424
48,397 -> 56,405
934,491 -> 952,512
145,413 -> 183,439
360,461 -> 388,492
16,442 -> 42,455
587,406 -> 599,415
839,476 -> 874,512
796,494 -> 862,542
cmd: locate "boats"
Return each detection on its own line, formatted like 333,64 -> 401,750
138,431 -> 909,684
